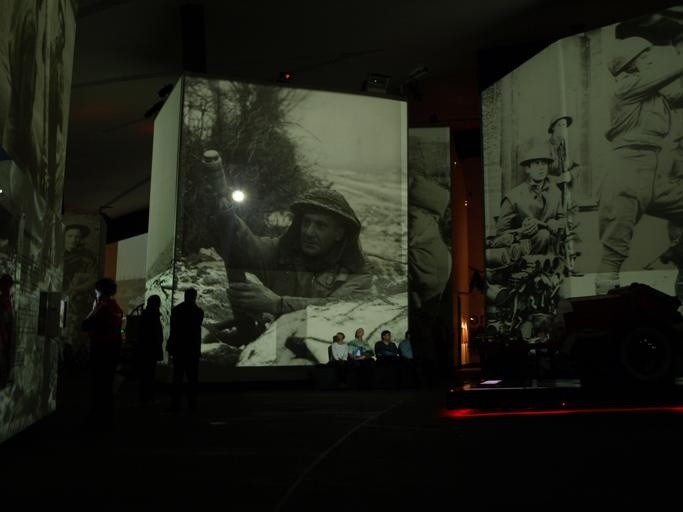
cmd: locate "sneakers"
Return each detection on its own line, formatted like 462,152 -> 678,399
572,271 -> 584,276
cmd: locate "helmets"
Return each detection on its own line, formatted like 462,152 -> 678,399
549,113 -> 573,132
609,36 -> 649,76
289,189 -> 360,225
519,148 -> 553,165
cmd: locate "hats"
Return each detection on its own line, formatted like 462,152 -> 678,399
408,175 -> 450,215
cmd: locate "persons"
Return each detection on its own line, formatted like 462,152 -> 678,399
331,328 -> 422,367
545,111 -> 584,277
496,146 -> 567,256
407,143 -> 454,309
76,278 -> 204,396
432,316 -> 450,380
62,224 -> 98,295
202,182 -> 373,315
593,36 -> 683,314
